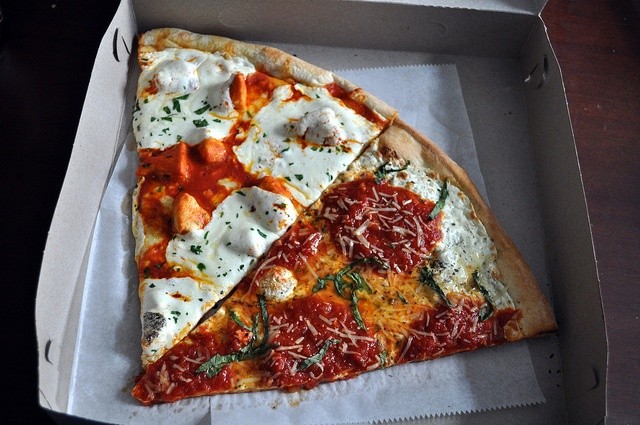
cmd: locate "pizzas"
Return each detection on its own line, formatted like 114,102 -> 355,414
129,116 -> 561,407
130,25 -> 398,375
